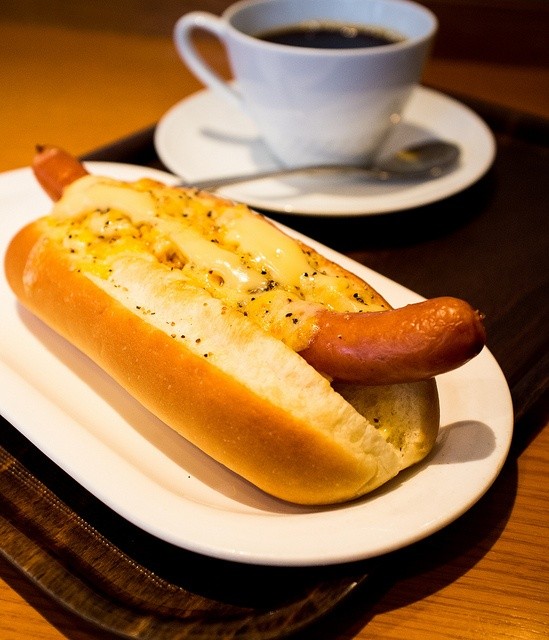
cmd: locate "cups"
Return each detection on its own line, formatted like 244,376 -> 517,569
173,0 -> 436,167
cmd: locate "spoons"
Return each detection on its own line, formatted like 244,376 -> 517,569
163,137 -> 462,192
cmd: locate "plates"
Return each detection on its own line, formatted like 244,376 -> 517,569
154,78 -> 497,218
1,161 -> 515,569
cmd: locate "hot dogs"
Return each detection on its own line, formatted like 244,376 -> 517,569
4,144 -> 487,504
